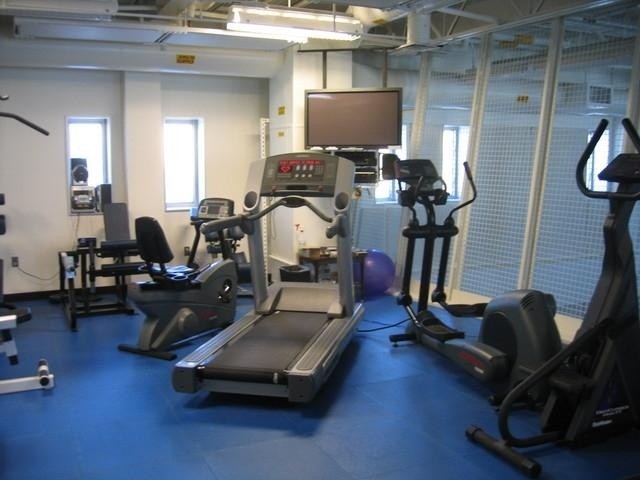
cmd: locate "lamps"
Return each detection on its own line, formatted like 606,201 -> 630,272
226,4 -> 363,41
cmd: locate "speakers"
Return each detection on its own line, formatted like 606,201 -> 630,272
95,184 -> 111,212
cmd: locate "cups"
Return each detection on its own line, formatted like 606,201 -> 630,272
191,207 -> 199,221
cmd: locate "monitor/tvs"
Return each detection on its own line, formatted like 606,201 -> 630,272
303,87 -> 401,148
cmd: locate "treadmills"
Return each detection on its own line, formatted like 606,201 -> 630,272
171,152 -> 366,403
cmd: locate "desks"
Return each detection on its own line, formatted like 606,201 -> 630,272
297,247 -> 368,295
